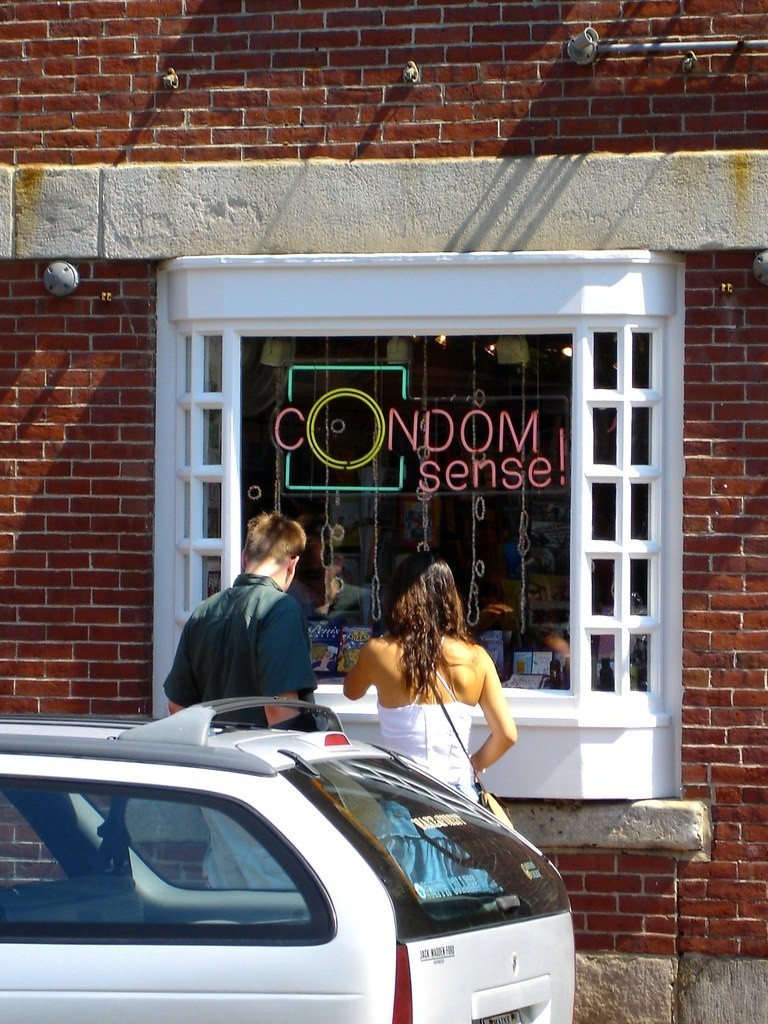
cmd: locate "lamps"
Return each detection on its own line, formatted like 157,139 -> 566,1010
497,335 -> 530,364
259,337 -> 293,367
387,336 -> 409,364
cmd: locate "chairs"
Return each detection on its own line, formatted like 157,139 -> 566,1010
0,875 -> 144,923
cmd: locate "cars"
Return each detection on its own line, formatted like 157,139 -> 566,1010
0,697 -> 575,1024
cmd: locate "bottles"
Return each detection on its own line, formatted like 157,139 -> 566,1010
599,658 -> 614,692
630,658 -> 640,691
562,656 -> 570,690
550,660 -> 562,689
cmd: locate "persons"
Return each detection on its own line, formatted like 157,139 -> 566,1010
163,509 -> 329,888
341,555 -> 516,900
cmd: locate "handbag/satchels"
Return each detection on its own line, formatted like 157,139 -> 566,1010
479,790 -> 513,829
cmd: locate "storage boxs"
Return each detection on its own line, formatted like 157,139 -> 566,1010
305,622 -> 376,675
